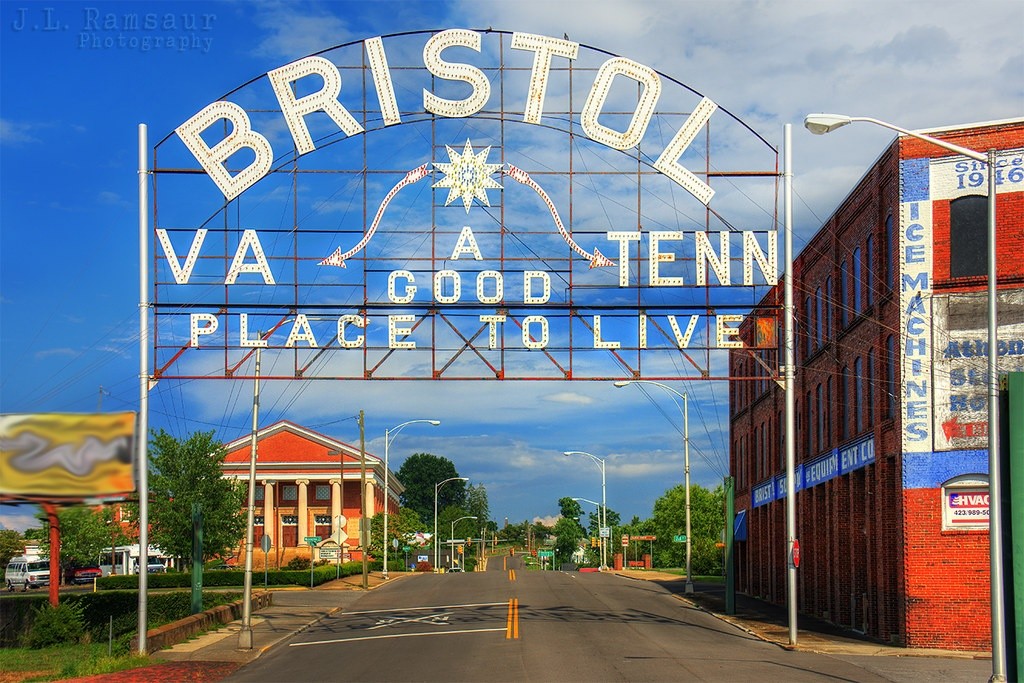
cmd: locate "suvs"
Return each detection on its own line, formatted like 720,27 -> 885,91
64,560 -> 103,586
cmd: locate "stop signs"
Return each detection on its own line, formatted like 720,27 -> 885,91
793,539 -> 800,567
620,535 -> 629,546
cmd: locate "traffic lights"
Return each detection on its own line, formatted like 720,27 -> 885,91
591,536 -> 596,548
458,546 -> 462,553
597,539 -> 600,547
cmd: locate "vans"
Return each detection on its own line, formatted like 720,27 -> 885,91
6,557 -> 50,592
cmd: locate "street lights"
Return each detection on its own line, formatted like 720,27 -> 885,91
381,420 -> 440,579
434,478 -> 468,572
615,380 -> 694,593
805,113 -> 1007,682
451,517 -> 477,568
237,317 -> 371,650
571,498 -> 603,566
564,452 -> 610,572
573,516 -> 613,570
